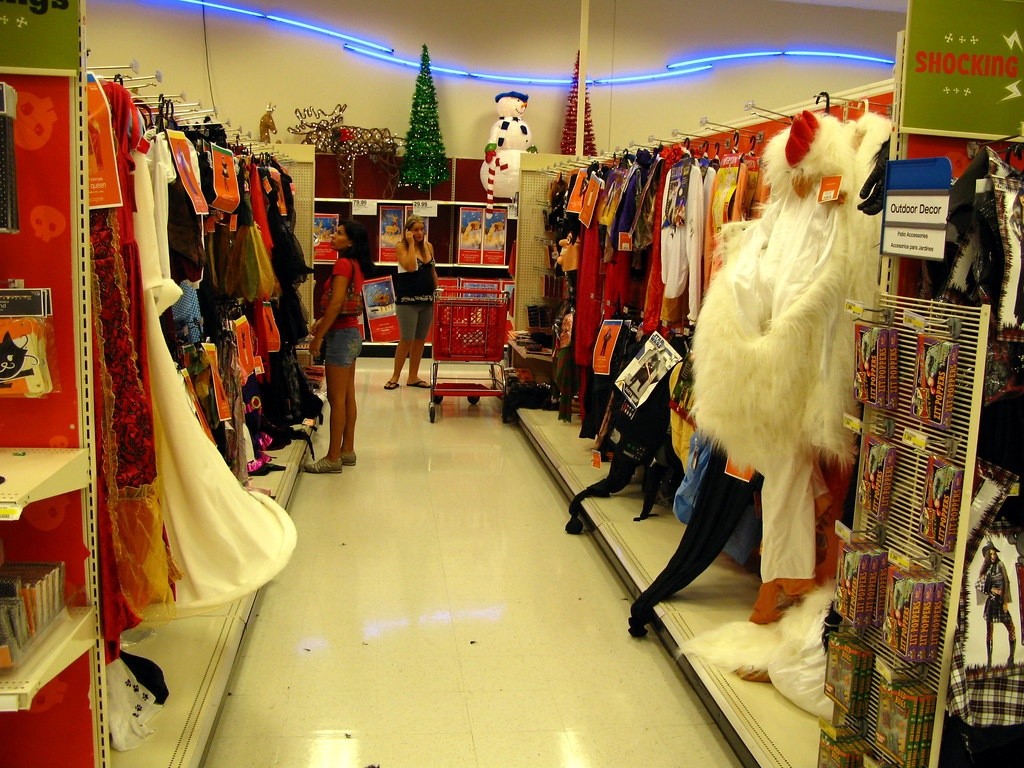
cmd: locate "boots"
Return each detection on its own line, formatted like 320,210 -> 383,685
1007,640 -> 1016,669
985,640 -> 993,672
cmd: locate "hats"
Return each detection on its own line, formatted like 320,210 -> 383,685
982,541 -> 1001,558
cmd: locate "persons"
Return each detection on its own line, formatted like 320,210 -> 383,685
304,220 -> 364,474
384,214 -> 440,390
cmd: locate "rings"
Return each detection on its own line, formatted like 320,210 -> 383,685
314,351 -> 316,353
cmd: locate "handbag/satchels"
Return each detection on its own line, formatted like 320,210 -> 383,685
326,256 -> 363,316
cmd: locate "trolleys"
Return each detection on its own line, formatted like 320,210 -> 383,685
427,288 -> 511,424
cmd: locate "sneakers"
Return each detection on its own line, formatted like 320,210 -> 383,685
340,450 -> 356,465
304,457 -> 342,473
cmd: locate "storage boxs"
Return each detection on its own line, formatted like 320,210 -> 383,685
424,277 -> 514,345
456,207 -> 508,266
314,214 -> 340,261
379,206 -> 403,264
405,206 -> 429,242
818,321 -> 967,768
357,276 -> 401,343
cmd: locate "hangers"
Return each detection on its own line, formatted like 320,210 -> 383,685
114,73 -> 274,168
176,299 -> 247,362
606,92 -> 870,172
1004,143 -> 1023,180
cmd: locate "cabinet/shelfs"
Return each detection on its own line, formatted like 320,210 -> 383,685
0,447 -> 97,711
313,197 -> 519,347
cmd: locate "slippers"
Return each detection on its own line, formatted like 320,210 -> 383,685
384,381 -> 399,389
407,381 -> 431,388
